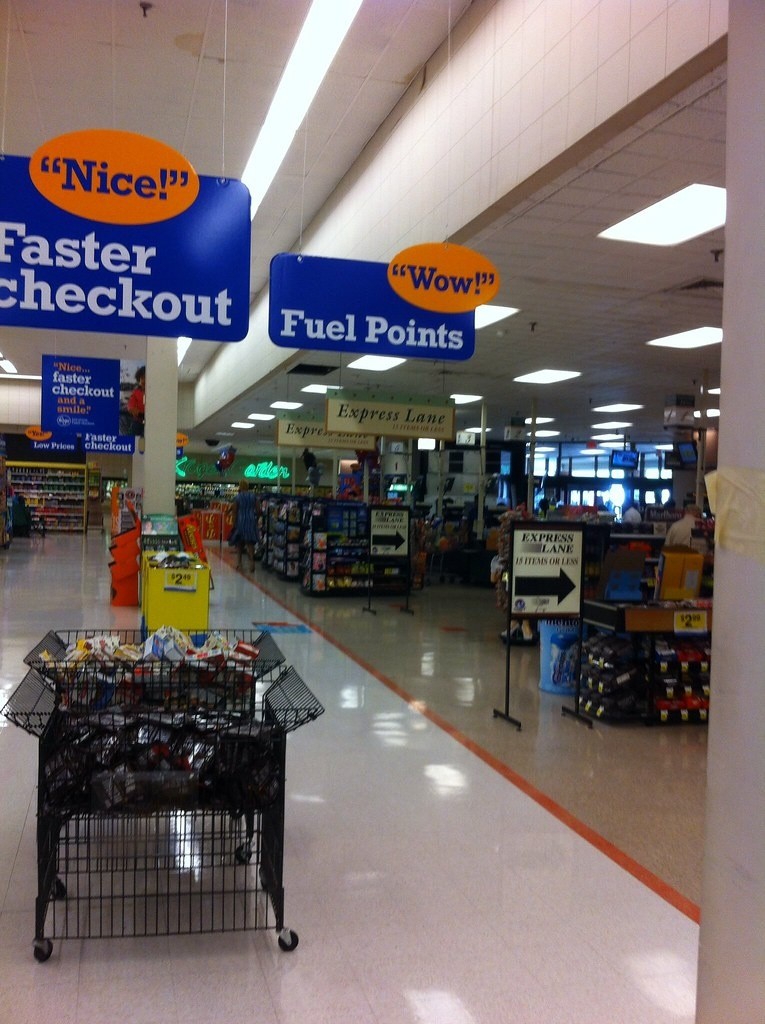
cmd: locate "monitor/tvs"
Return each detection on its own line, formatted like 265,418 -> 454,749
676,441 -> 698,464
611,450 -> 640,470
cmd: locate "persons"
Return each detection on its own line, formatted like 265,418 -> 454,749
622,501 -> 642,524
13,493 -> 19,505
539,499 -> 557,518
127,366 -> 145,434
225,478 -> 261,573
470,494 -> 487,520
664,505 -> 700,545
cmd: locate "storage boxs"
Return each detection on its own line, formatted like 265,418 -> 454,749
653,545 -> 704,601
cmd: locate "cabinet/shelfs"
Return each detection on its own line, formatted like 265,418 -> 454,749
4,461 -> 90,535
173,480 -> 412,597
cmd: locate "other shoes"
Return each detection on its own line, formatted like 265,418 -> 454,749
250,564 -> 255,573
235,563 -> 242,570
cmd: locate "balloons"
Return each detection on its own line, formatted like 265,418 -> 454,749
215,446 -> 235,472
306,467 -> 323,489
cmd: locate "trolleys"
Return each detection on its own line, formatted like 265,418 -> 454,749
0,628 -> 325,963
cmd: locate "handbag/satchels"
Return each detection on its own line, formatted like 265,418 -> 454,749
227,528 -> 241,546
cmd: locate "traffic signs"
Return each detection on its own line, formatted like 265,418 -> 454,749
368,505 -> 412,566
510,519 -> 587,620
